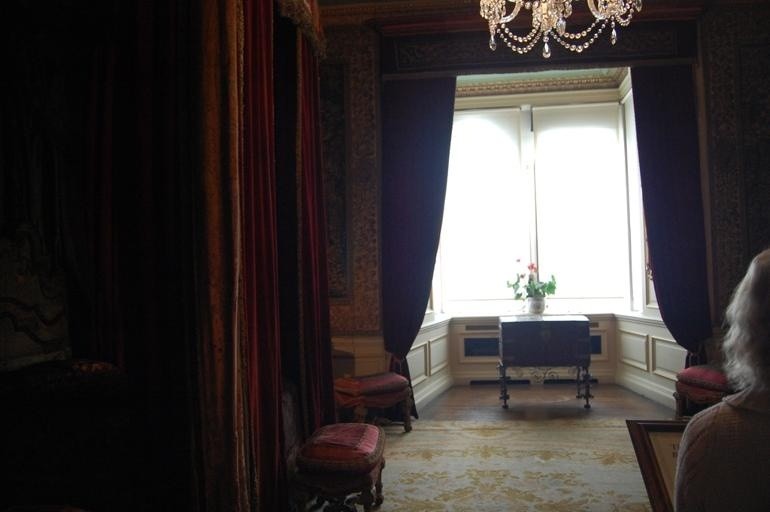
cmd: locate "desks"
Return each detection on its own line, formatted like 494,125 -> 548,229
496,312 -> 594,409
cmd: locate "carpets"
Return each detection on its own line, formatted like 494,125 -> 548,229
304,416 -> 691,511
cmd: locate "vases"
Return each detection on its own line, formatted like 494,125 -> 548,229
527,291 -> 547,316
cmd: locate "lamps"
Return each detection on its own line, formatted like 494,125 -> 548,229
477,1 -> 647,60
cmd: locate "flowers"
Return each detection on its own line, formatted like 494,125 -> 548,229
502,257 -> 560,299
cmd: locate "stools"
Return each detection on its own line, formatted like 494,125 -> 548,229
669,363 -> 731,418
290,422 -> 389,511
334,371 -> 414,432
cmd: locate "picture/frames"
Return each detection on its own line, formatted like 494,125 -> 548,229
626,415 -> 691,511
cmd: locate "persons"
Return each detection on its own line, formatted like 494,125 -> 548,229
678,246 -> 770,510
